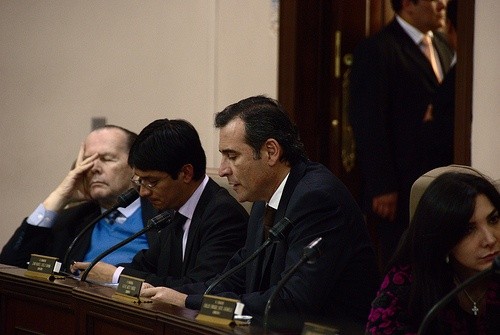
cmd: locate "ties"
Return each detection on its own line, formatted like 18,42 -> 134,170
264,204 -> 276,242
422,36 -> 440,121
172,211 -> 188,263
105,210 -> 120,225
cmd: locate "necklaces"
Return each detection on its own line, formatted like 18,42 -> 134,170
457,277 -> 491,315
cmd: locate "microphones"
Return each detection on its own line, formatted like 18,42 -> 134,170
52,211 -> 174,288
234,236 -> 325,334
52,186 -> 139,277
201,216 -> 292,304
417,255 -> 500,334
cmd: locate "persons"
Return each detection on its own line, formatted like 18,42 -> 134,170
365,165 -> 500,335
140,96 -> 377,322
73,118 -> 250,287
346,0 -> 457,254
0,125 -> 165,268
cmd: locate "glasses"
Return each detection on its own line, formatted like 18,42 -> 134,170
129,174 -> 169,189
